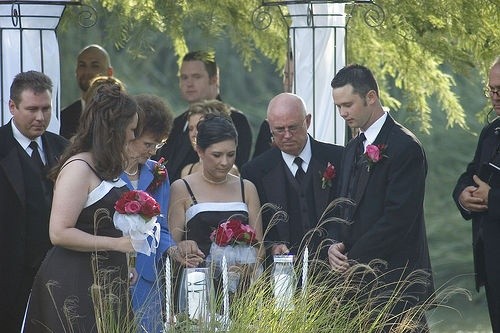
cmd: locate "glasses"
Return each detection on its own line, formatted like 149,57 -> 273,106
143,143 -> 164,150
483,86 -> 500,98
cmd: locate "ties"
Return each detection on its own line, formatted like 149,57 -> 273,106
294,157 -> 306,184
355,133 -> 365,162
29,141 -> 45,170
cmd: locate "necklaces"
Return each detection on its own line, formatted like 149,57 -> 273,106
123,167 -> 138,176
203,175 -> 228,185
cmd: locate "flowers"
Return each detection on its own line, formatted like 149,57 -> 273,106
210,220 -> 262,293
145,157 -> 170,195
321,161 -> 336,191
113,189 -> 165,269
359,145 -> 390,173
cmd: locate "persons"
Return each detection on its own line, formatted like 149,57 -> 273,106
241,92 -> 345,270
181,98 -> 240,179
252,51 -> 293,159
150,50 -> 252,186
0,71 -> 75,333
20,76 -> 147,333
118,93 -> 202,333
324,65 -> 438,333
453,59 -> 500,333
60,44 -> 113,143
169,111 -> 264,315
